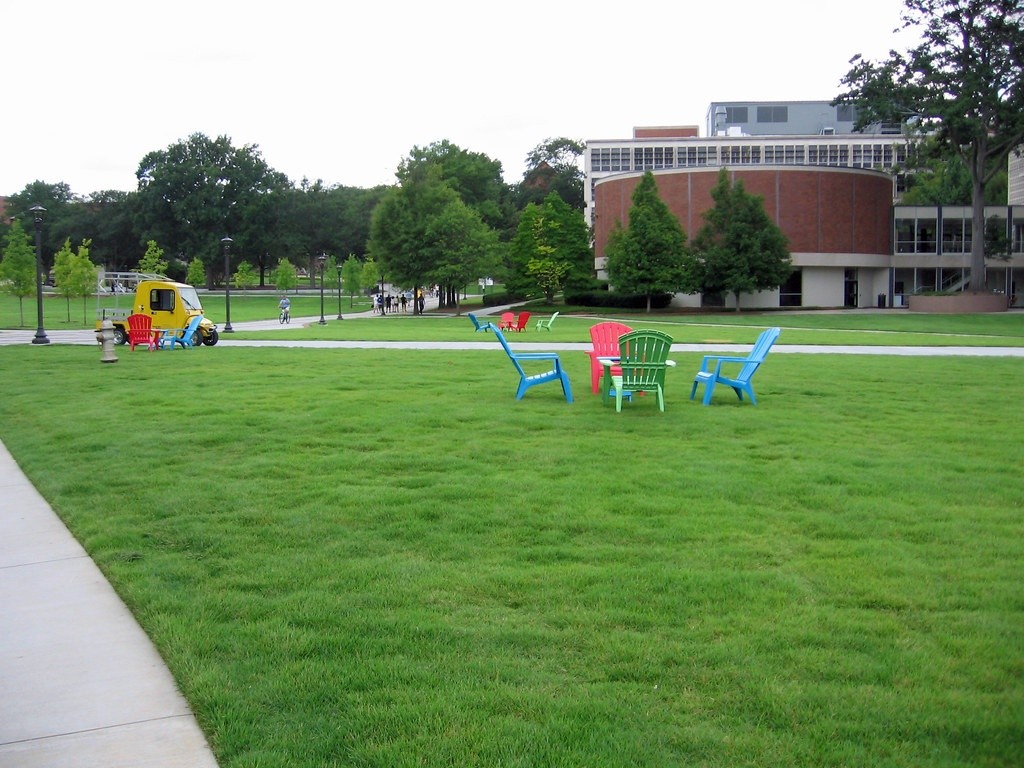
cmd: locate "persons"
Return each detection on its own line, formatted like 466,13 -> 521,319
278,295 -> 290,322
418,294 -> 425,315
372,292 -> 407,313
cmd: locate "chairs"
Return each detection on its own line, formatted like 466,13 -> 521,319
601,328 -> 674,414
587,322 -> 636,394
498,312 -> 515,331
468,313 -> 491,334
535,311 -> 560,331
163,314 -> 203,350
127,314 -> 153,352
687,325 -> 782,406
509,311 -> 531,332
487,322 -> 574,403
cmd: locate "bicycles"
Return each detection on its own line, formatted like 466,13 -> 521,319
278,306 -> 291,324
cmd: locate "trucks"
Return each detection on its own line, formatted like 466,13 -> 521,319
95,280 -> 218,347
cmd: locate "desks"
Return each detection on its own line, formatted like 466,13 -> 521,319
597,353 -> 679,406
148,329 -> 170,351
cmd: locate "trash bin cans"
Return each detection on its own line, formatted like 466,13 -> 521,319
878,293 -> 886,308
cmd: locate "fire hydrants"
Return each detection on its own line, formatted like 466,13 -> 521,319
96,316 -> 119,363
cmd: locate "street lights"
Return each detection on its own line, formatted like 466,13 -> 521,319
27,201 -> 50,344
336,265 -> 342,320
317,255 -> 326,325
220,234 -> 235,333
